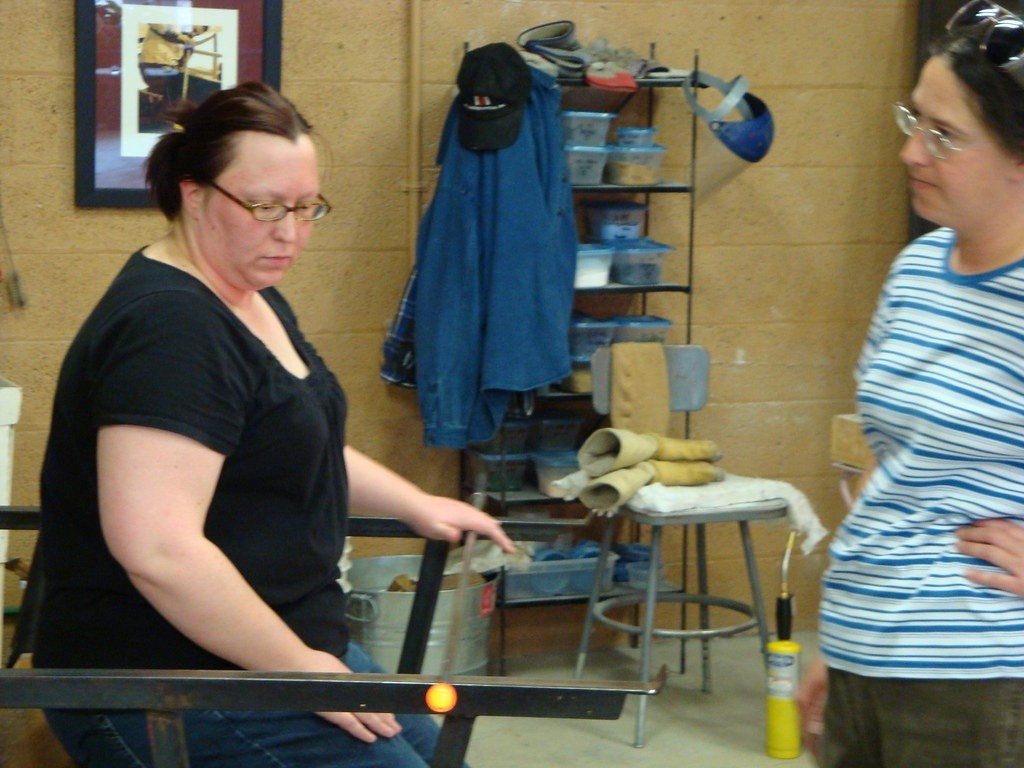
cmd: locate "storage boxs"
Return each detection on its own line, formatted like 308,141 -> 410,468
461,111 -> 678,601
344,550 -> 501,674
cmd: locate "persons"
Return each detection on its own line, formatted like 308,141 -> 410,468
9,78 -> 518,768
819,23 -> 1024,768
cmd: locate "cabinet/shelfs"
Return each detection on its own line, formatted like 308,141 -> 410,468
460,43 -> 701,675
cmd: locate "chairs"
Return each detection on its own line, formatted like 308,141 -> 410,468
576,346 -> 789,748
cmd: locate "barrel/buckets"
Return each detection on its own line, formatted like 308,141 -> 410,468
342,553 -> 499,677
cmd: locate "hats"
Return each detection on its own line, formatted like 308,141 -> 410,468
457,43 -> 530,152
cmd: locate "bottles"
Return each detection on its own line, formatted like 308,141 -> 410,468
765,596 -> 803,762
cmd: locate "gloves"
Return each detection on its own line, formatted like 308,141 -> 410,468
575,430 -> 726,518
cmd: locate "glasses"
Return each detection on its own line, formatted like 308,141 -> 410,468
199,178 -> 332,224
944,0 -> 1024,69
892,102 -> 1005,162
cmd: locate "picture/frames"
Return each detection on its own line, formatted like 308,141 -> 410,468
75,0 -> 283,207
